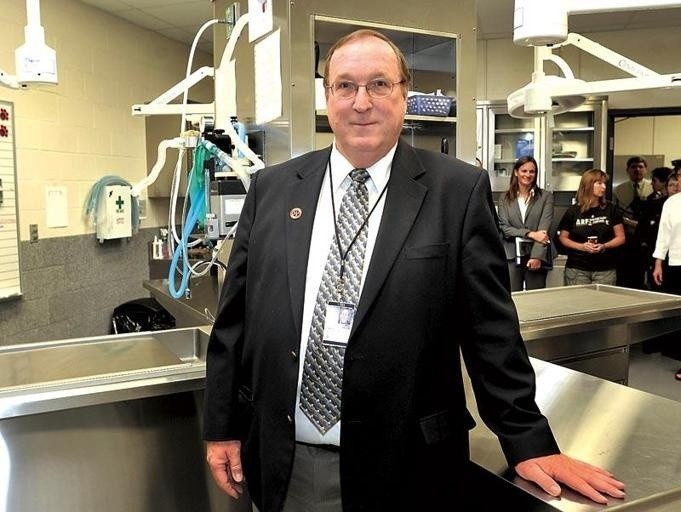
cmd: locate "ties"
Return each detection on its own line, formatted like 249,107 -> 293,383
298,166 -> 371,436
630,183 -> 642,201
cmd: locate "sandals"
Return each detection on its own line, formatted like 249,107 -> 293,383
675,367 -> 681,382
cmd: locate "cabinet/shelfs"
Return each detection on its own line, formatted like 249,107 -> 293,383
477,94 -> 607,193
264,12 -> 479,166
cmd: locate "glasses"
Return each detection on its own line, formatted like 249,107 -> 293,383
320,79 -> 405,98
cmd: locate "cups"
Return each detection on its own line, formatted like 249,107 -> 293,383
588,237 -> 597,244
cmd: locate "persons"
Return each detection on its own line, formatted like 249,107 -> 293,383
200,29 -> 627,512
613,155 -> 652,205
651,166 -> 667,196
649,170 -> 677,276
499,155 -> 554,292
558,168 -> 627,289
653,167 -> 681,382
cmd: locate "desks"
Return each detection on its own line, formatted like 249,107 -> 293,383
143,276 -> 217,326
511,285 -> 681,385
458,346 -> 681,508
0,326 -> 251,512
